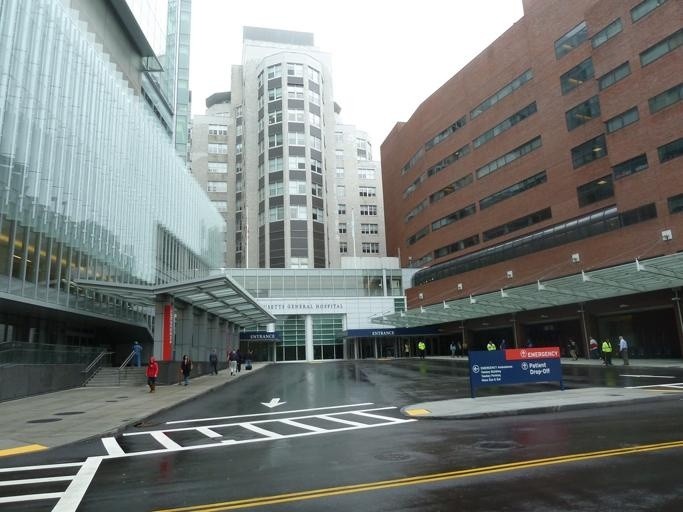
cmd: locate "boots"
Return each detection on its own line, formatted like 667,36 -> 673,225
150,384 -> 155,392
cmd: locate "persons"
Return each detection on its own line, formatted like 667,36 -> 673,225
588,335 -> 602,360
418,339 -> 426,359
404,341 -> 408,358
225,344 -> 254,376
145,356 -> 158,392
179,354 -> 193,386
566,338 -> 577,361
130,341 -> 145,367
486,340 -> 496,351
601,337 -> 613,366
499,338 -> 507,351
617,335 -> 630,365
449,340 -> 465,358
525,338 -> 533,347
206,348 -> 218,376
